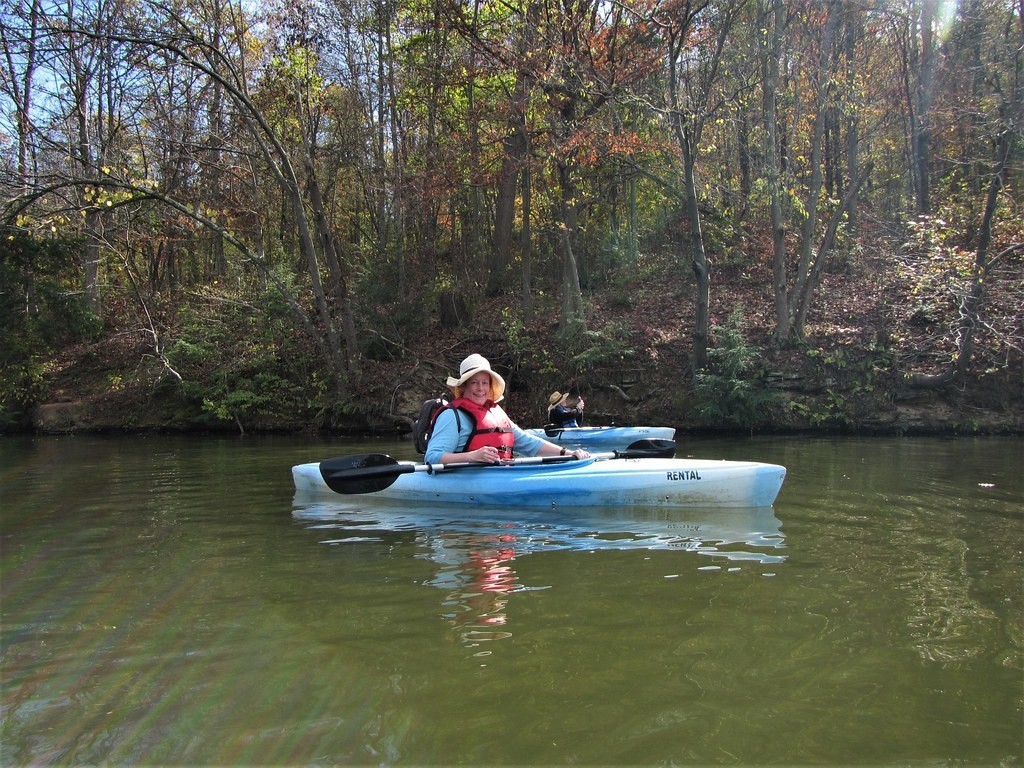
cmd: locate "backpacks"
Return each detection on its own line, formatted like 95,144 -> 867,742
413,398 -> 460,454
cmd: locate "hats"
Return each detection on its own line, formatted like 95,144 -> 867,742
446,354 -> 505,402
547,391 -> 569,410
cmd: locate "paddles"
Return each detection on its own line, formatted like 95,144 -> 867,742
317,437 -> 678,495
543,424 -> 618,439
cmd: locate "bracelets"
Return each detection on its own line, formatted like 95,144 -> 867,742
560,447 -> 566,455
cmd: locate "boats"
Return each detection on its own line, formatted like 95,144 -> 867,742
291,449 -> 786,512
522,426 -> 675,452
288,508 -> 787,563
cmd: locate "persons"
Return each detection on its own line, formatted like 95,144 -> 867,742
547,390 -> 585,430
425,353 -> 592,475
